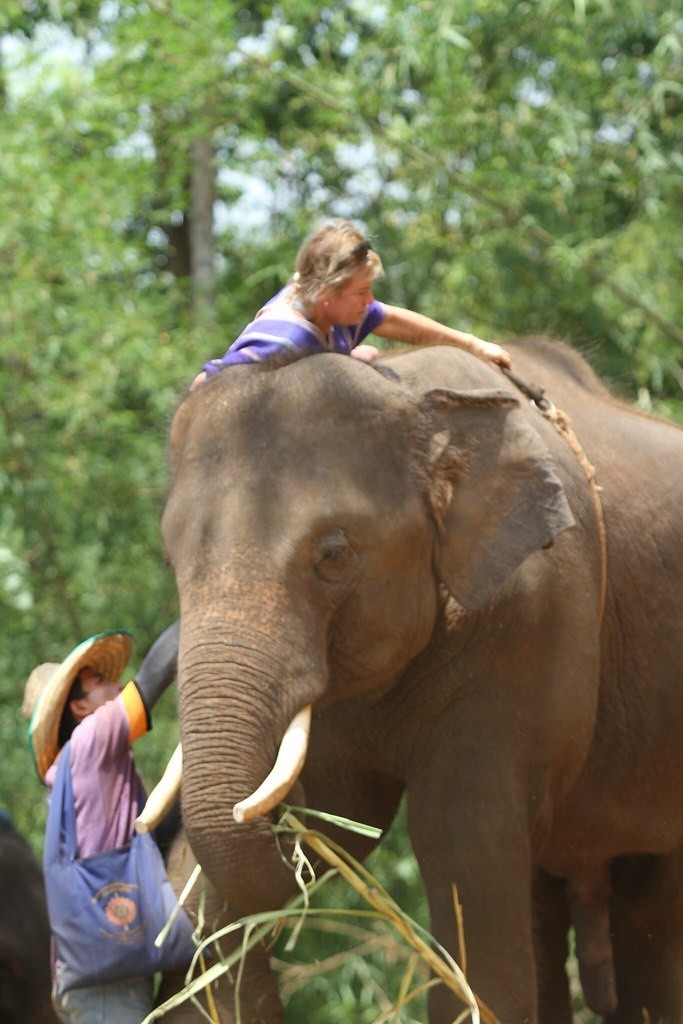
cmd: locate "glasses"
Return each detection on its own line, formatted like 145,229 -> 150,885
335,240 -> 371,272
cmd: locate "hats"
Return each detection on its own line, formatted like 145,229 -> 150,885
21,628 -> 134,790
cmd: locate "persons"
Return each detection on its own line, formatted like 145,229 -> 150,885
21,619 -> 182,1024
186,222 -> 512,392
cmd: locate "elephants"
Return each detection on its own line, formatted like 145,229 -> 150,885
157,325 -> 683,1024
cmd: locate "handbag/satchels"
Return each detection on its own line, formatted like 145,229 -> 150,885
42,744 -> 213,998
